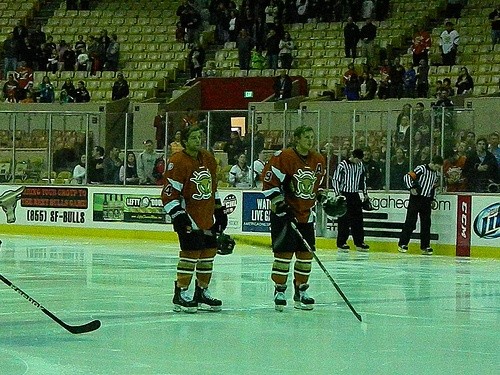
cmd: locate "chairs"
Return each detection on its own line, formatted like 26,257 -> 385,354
0,0 -> 500,183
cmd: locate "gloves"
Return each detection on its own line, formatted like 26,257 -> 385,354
209,199 -> 228,234
275,204 -> 293,225
168,204 -> 192,236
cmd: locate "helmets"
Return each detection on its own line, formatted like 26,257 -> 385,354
215,233 -> 235,256
320,196 -> 348,220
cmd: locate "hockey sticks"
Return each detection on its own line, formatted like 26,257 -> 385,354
0,274 -> 102,335
290,221 -> 363,322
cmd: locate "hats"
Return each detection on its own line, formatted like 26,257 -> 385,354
361,198 -> 373,211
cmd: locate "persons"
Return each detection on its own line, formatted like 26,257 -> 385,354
332,150 -> 371,249
229,152 -> 253,189
112,72 -> 129,100
154,108 -> 172,149
226,124 -> 264,165
436,89 -> 454,127
182,108 -> 198,130
322,102 -> 500,191
403,156 -> 444,252
3,23 -> 120,103
162,126 -> 229,306
252,146 -> 267,188
163,0 -> 500,100
72,130 -> 182,184
199,114 -> 215,142
262,124 -> 333,303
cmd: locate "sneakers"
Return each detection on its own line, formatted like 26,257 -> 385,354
356,242 -> 370,252
337,243 -> 350,253
397,243 -> 408,253
421,246 -> 434,255
273,282 -> 289,312
172,280 -> 198,314
292,279 -> 315,311
193,279 -> 223,311
